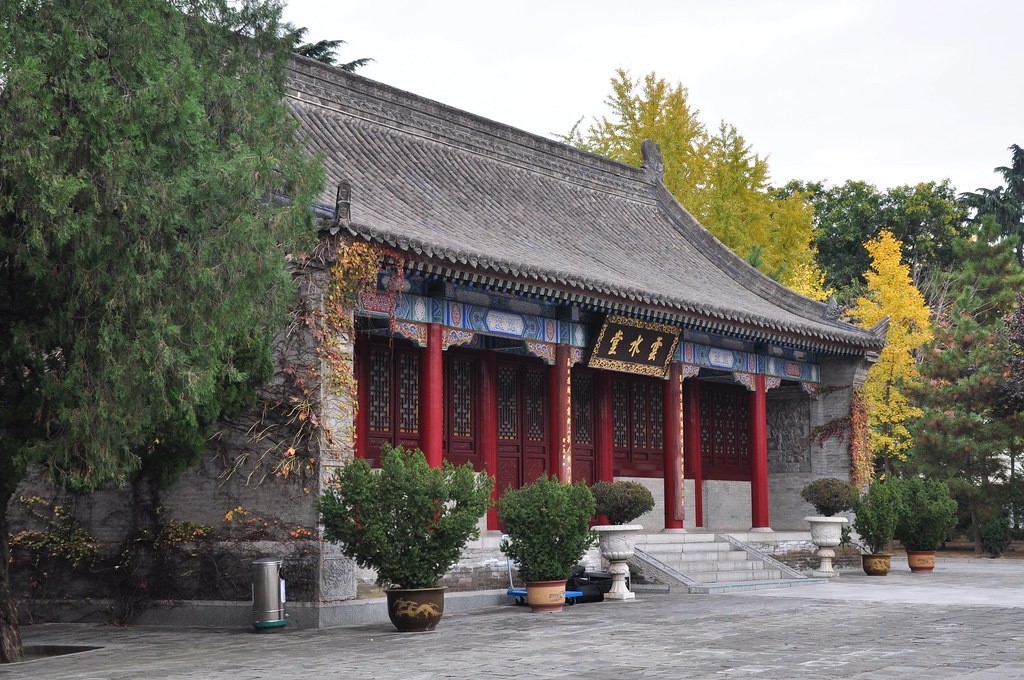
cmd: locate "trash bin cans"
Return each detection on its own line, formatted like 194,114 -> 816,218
252,558 -> 288,633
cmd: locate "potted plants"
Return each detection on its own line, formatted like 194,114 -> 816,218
310,441 -> 496,632
891,474 -> 959,573
493,469 -> 597,613
837,476 -> 904,576
799,478 -> 860,545
588,480 -> 656,560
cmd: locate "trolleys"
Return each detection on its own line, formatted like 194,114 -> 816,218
502,534 -> 583,606
